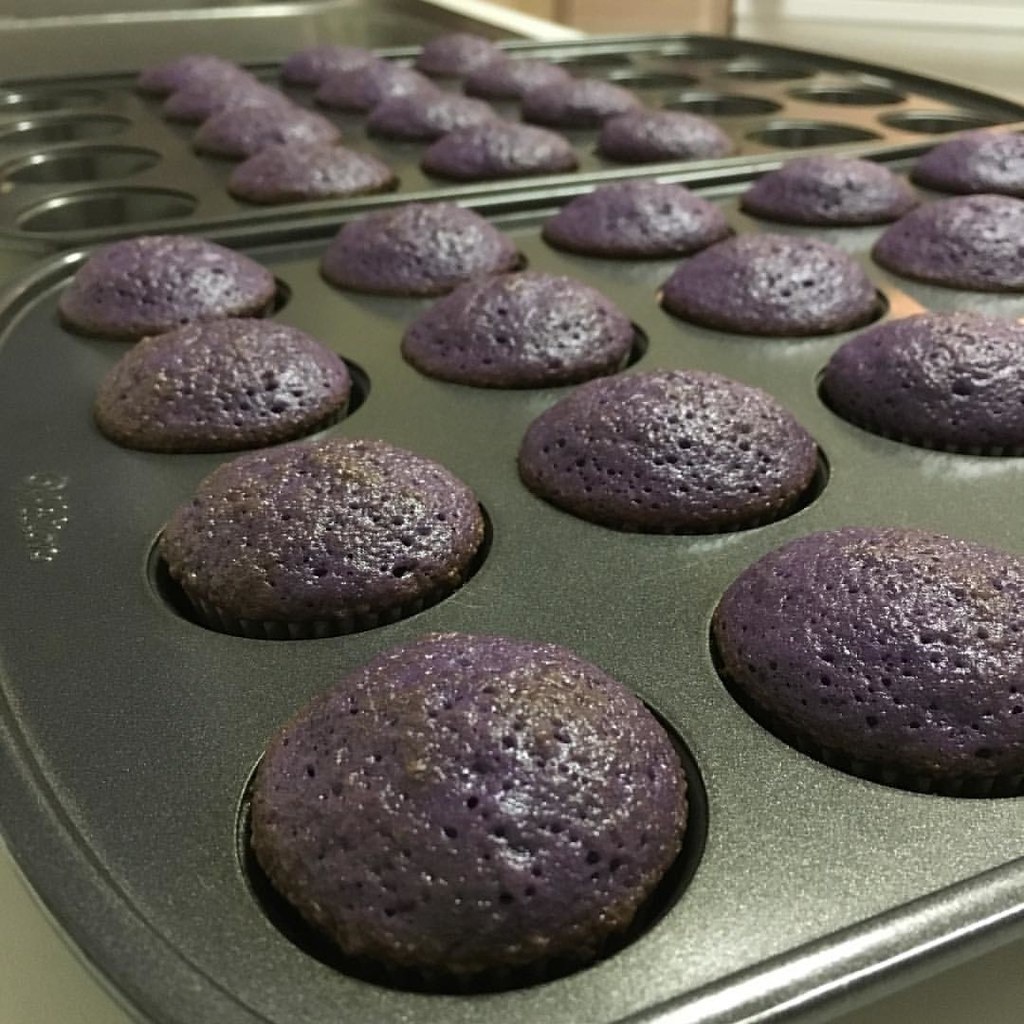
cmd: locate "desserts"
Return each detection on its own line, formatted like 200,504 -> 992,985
61,38 -> 1022,986
